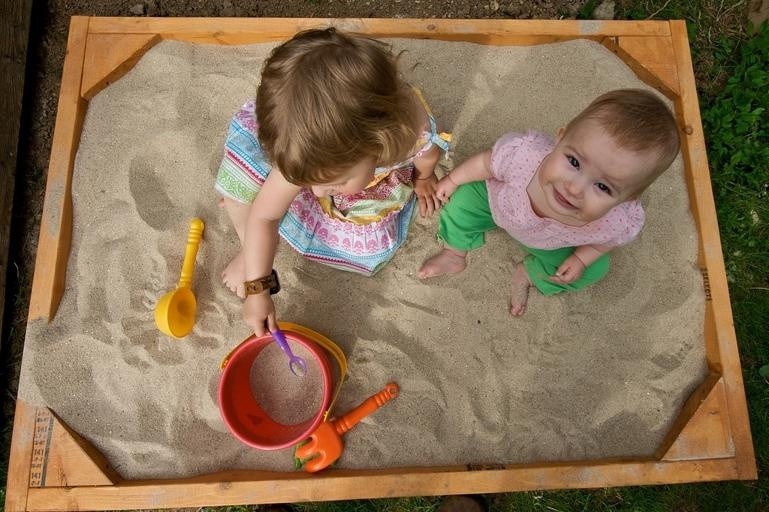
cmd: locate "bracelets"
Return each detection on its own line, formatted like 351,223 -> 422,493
241,270 -> 283,298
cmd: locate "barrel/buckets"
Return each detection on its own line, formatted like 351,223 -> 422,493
219,322 -> 348,451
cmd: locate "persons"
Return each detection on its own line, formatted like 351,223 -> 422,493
213,23 -> 457,341
417,87 -> 682,316
